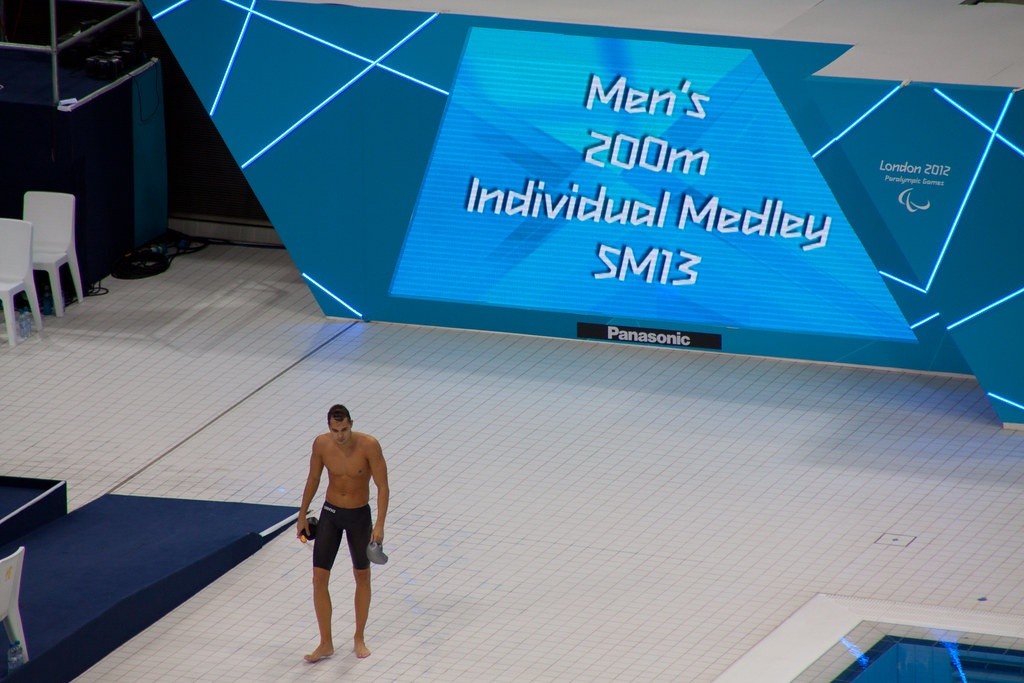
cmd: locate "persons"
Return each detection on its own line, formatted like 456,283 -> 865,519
297,404 -> 389,663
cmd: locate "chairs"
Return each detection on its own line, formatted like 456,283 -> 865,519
0,218 -> 43,346
23,192 -> 84,318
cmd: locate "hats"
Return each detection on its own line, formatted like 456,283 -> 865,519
366,542 -> 388,564
